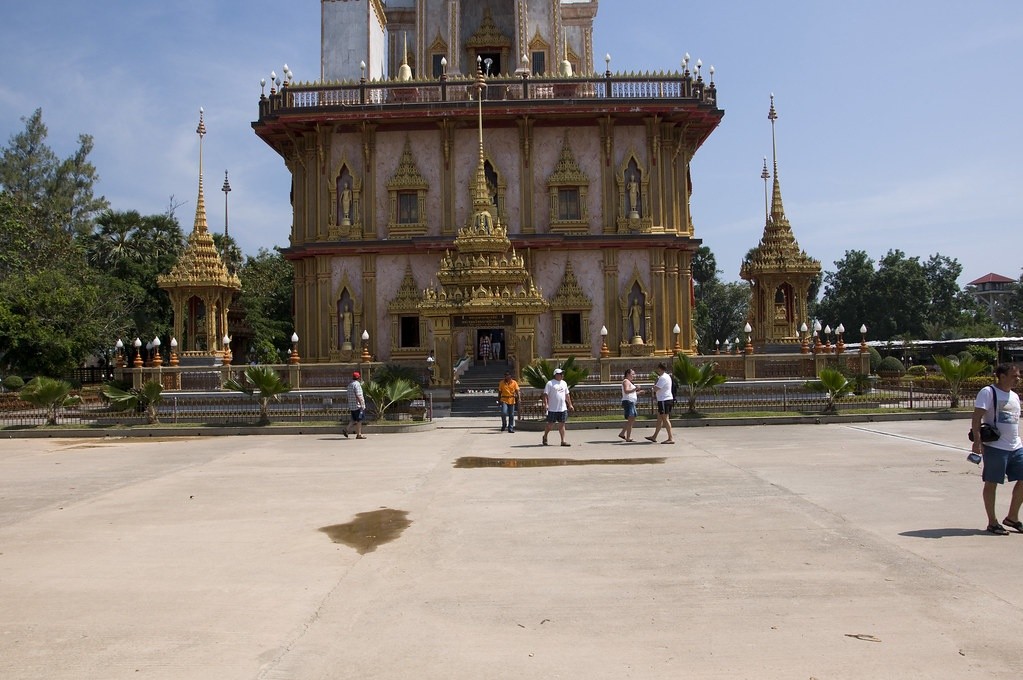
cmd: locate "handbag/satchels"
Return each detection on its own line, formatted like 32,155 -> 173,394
969,423 -> 1001,442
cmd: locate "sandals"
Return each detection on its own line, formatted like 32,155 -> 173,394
987,523 -> 1009,535
1002,517 -> 1023,533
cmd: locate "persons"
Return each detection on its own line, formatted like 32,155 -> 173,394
969,363 -> 1023,535
342,372 -> 367,439
626,174 -> 640,210
629,297 -> 643,336
497,371 -> 521,434
340,305 -> 352,342
646,363 -> 677,445
619,368 -> 645,444
479,331 -> 502,366
541,367 -> 574,447
339,183 -> 353,218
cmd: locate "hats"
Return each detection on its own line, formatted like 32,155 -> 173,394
353,372 -> 360,378
553,369 -> 565,375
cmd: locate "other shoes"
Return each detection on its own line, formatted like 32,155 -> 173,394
645,436 -> 657,442
543,435 -> 548,445
626,439 -> 635,442
561,442 -> 570,446
342,429 -> 349,438
356,435 -> 366,439
618,434 -> 626,440
501,425 -> 506,431
508,430 -> 515,433
660,440 -> 675,444
